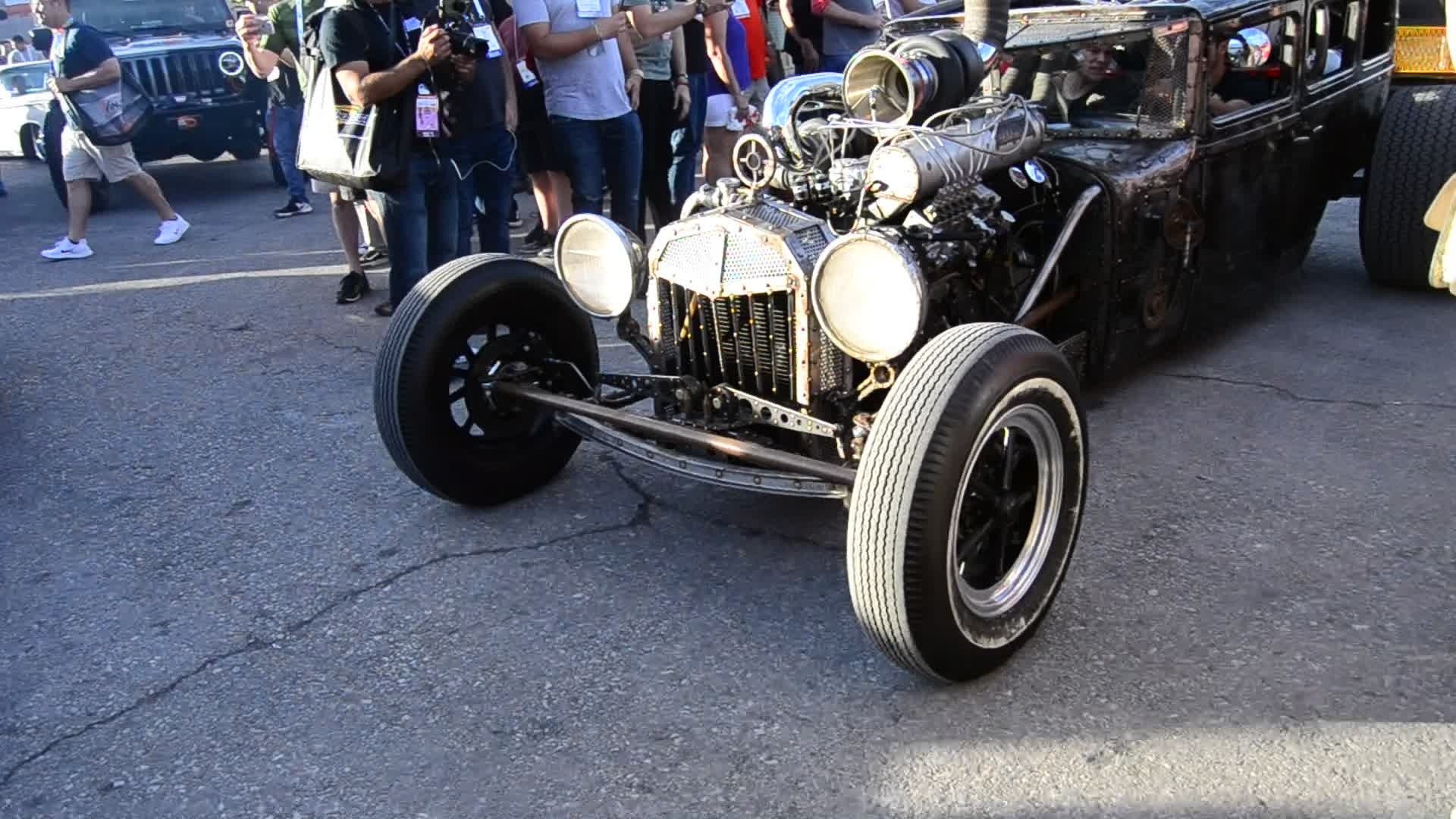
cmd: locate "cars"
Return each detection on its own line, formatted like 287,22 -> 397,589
1,61 -> 62,163
370,0 -> 1455,688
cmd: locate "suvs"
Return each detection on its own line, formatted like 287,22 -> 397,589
42,2 -> 308,209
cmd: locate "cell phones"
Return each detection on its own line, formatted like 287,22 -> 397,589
254,16 -> 276,35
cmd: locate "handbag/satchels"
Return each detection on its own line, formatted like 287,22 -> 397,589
56,64 -> 153,145
294,60 -> 398,189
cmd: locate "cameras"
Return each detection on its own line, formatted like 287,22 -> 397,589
439,20 -> 489,63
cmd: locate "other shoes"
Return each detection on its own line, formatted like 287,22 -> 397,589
517,235 -> 553,257
376,300 -> 396,316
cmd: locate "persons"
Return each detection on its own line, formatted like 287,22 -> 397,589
31,0 -> 192,261
233,0 -> 966,318
0,24 -> 47,66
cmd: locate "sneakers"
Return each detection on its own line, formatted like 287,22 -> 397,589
153,213 -> 189,244
40,237 -> 94,258
336,271 -> 370,303
274,197 -> 315,218
